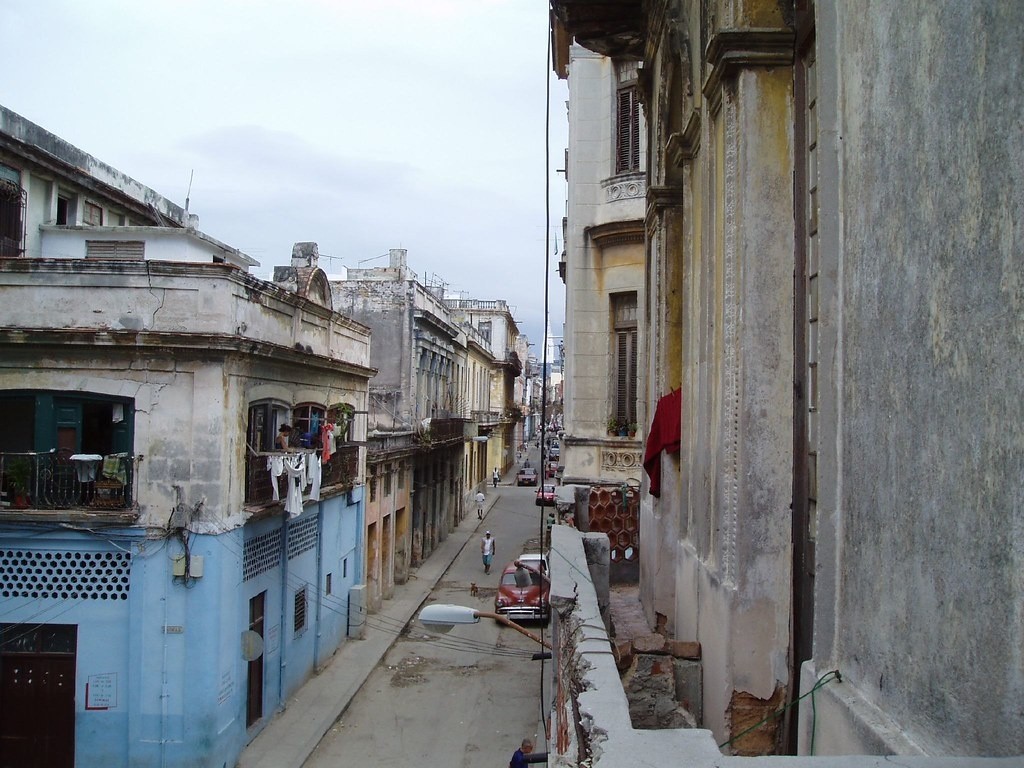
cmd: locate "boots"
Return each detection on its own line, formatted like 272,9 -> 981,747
484,564 -> 490,575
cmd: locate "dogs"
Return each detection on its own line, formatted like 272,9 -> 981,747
470,583 -> 478,597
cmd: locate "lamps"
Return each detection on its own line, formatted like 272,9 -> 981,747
419,603 -> 562,660
446,436 -> 487,447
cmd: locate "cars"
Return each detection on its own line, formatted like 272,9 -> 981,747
546,463 -> 558,477
516,468 -> 538,487
516,554 -> 549,577
549,448 -> 559,461
534,484 -> 555,508
493,561 -> 551,629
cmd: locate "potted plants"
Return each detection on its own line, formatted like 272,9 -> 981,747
6,455 -> 35,509
606,416 -> 637,437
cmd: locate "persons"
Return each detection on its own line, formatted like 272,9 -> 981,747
276,423 -> 292,449
544,447 -> 547,459
524,459 -> 530,468
524,441 -> 528,452
481,531 -> 495,575
475,491 -> 485,520
493,467 -> 499,488
536,441 -> 539,449
545,513 -> 555,548
554,442 -> 559,448
547,438 -> 550,450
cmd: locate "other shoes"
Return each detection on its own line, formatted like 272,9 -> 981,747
478,516 -> 482,519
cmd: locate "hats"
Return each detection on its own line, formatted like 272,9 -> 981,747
485,530 -> 491,534
